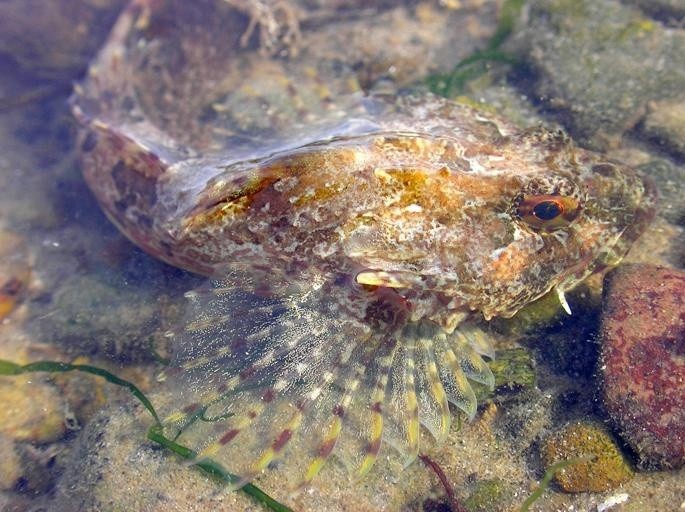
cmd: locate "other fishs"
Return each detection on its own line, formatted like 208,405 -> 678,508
63,0 -> 662,505
